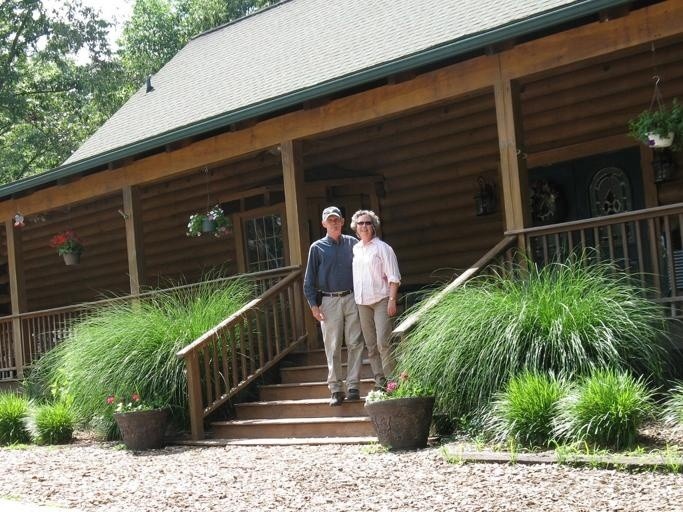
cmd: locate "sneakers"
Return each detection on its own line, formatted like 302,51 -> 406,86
348,389 -> 359,400
329,392 -> 345,406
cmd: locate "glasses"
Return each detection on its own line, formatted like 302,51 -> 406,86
357,222 -> 372,225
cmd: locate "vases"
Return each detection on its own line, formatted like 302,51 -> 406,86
200,216 -> 213,236
364,398 -> 432,447
117,410 -> 169,450
63,247 -> 79,269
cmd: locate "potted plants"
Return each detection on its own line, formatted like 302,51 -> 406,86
626,98 -> 683,149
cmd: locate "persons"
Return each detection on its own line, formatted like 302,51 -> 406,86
348,209 -> 402,391
304,206 -> 361,407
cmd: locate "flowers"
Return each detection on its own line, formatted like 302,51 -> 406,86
102,392 -> 175,410
187,205 -> 226,238
359,377 -> 434,401
50,229 -> 84,254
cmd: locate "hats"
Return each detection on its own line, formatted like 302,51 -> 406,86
322,206 -> 342,221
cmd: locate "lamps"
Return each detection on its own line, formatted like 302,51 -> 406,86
473,173 -> 496,218
651,151 -> 675,185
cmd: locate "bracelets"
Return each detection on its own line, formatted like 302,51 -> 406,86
389,297 -> 396,301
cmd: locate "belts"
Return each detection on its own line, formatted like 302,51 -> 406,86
322,289 -> 353,297
